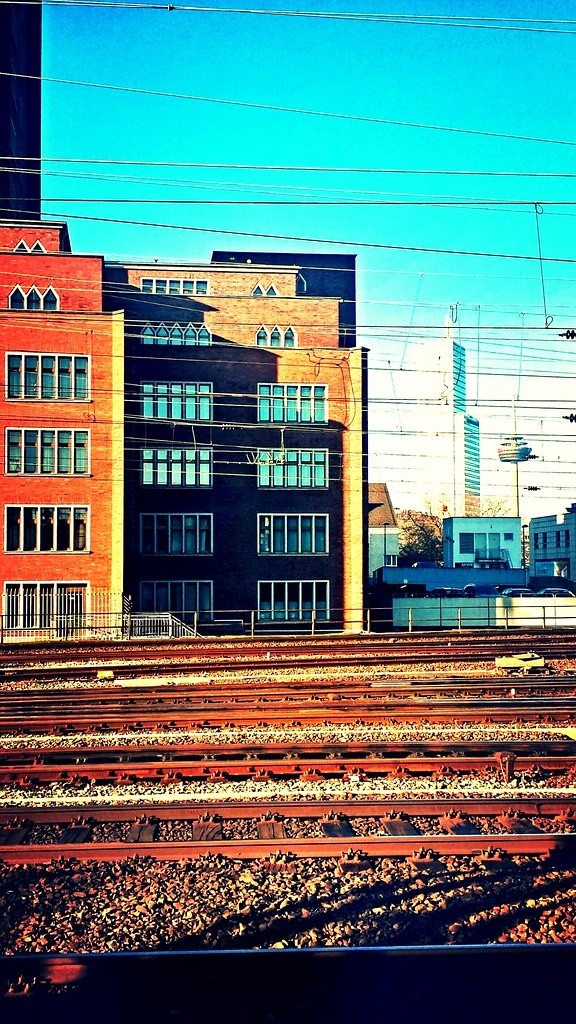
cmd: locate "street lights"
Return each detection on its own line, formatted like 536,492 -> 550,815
383,521 -> 390,567
521,524 -> 530,587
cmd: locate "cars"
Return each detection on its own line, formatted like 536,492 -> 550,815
412,583 -> 574,598
